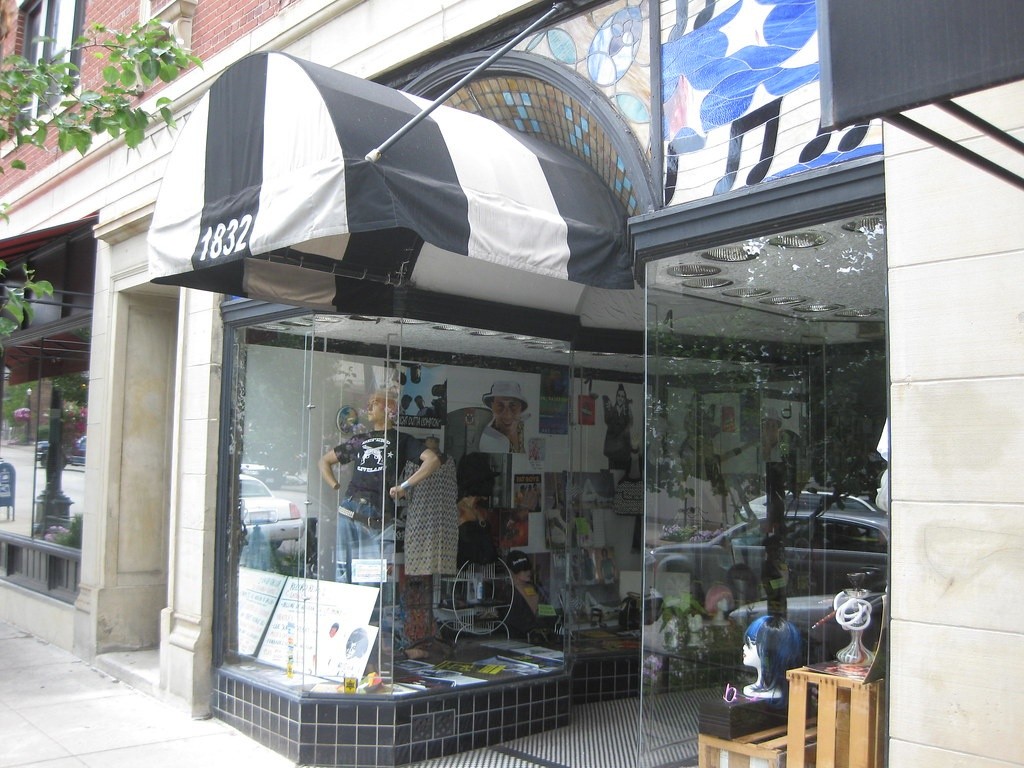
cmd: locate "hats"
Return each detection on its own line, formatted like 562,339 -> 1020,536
507,550 -> 532,574
482,381 -> 528,413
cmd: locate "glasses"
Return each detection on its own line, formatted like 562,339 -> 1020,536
491,402 -> 521,415
743,636 -> 756,648
723,684 -> 737,702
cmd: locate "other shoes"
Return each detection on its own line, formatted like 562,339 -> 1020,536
361,672 -> 382,690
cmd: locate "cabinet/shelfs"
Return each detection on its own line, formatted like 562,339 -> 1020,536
698,661 -> 889,768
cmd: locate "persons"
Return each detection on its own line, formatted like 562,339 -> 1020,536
318,389 -> 458,677
385,362 -> 448,416
743,615 -> 802,710
507,550 -> 560,640
480,380 -> 802,623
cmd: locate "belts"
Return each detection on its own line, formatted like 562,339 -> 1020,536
338,506 -> 391,529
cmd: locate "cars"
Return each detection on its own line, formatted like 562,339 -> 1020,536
738,490 -> 879,523
650,509 -> 888,610
725,591 -> 887,663
238,474 -> 304,553
36,435 -> 87,466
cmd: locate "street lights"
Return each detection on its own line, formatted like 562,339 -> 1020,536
26,385 -> 32,444
